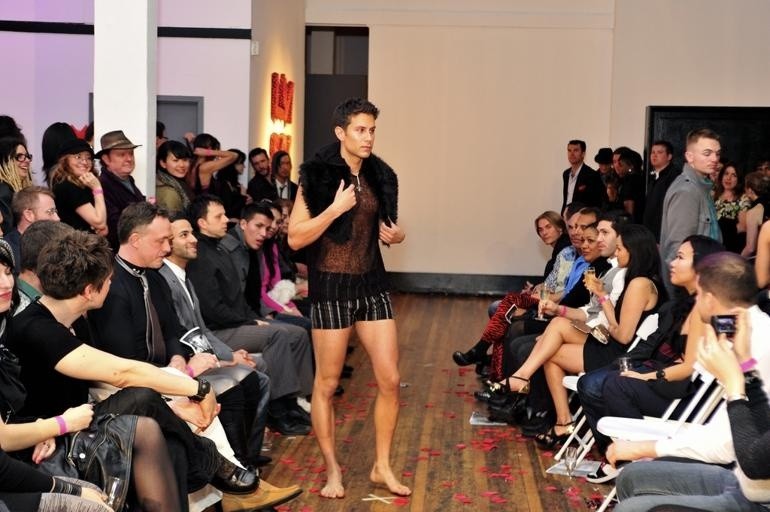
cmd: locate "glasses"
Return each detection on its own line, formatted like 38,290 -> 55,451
8,155 -> 33,162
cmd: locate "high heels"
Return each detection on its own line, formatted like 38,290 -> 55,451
453,347 -> 489,375
535,420 -> 579,445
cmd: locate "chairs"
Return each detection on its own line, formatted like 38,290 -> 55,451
555,310 -> 747,511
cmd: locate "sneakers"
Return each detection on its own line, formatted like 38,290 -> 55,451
586,457 -> 630,483
335,344 -> 353,395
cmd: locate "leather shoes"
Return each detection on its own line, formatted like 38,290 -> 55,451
207,456 -> 303,511
474,370 -> 555,437
264,403 -> 312,435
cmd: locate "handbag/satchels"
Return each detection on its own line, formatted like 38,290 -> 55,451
569,319 -> 611,345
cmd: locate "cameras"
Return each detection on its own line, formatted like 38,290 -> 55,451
711,313 -> 737,337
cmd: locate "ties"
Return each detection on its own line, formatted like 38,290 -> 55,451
280,186 -> 286,199
138,274 -> 166,367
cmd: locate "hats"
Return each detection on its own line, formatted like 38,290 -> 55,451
594,148 -> 613,165
62,139 -> 95,161
91,130 -> 143,158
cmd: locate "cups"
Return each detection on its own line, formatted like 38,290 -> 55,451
619,357 -> 633,373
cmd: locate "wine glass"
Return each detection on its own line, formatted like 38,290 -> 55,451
535,286 -> 551,322
584,267 -> 596,309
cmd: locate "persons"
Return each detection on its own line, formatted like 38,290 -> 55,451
454,128 -> 770,510
288,100 -> 412,498
0,116 -> 355,512
